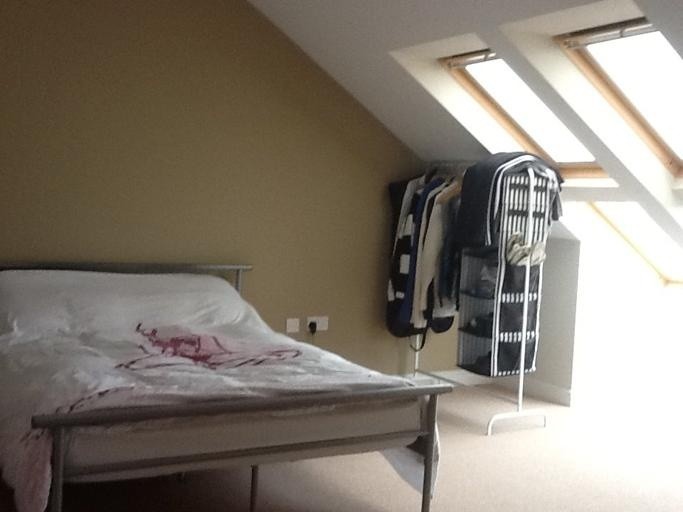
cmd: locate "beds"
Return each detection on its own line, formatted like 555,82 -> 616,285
0,259 -> 454,512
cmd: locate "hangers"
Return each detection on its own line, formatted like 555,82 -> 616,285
418,159 -> 466,187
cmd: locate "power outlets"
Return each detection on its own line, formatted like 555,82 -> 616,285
306,316 -> 329,332
